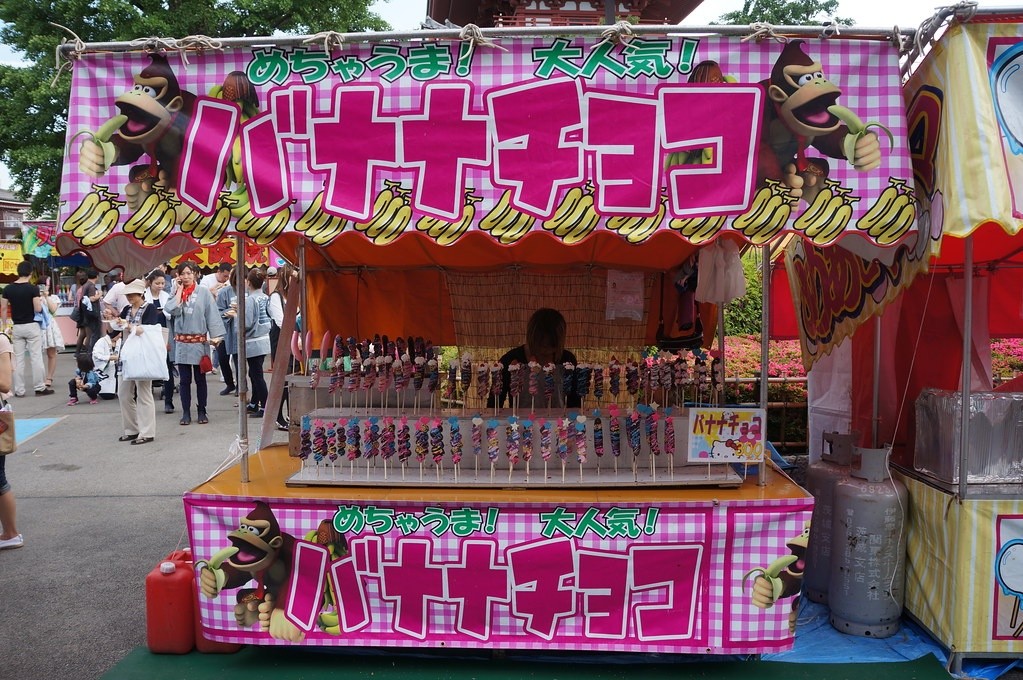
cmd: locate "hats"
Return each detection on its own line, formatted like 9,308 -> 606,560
118,279 -> 145,295
267,267 -> 277,275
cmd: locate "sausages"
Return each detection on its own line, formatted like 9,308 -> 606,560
291,331 -> 342,363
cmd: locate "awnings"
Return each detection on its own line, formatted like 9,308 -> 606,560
55,32 -> 919,488
763,8 -> 1023,498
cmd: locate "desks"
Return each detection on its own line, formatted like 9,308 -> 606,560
182,441 -> 811,663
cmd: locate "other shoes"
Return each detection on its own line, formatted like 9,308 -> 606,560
180,414 -> 191,424
67,397 -> 79,405
220,385 -> 235,395
35,388 -> 54,395
250,409 -> 264,417
119,434 -> 139,440
131,436 -> 154,445
197,405 -> 208,423
247,403 -> 258,413
45,378 -> 52,387
165,399 -> 174,413
275,420 -> 289,430
90,399 -> 97,404
15,393 -> 25,397
0,534 -> 24,549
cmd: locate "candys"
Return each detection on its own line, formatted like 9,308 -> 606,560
299,336 -> 724,466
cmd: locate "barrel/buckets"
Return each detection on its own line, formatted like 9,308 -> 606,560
145,550 -> 241,654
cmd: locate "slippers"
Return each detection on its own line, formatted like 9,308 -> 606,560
656,318 -> 704,353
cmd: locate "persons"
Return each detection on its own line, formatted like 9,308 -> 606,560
101,279 -> 162,447
68,254 -> 305,413
265,262 -> 303,431
164,261 -> 227,426
0,331 -> 27,548
235,267 -> 271,419
487,306 -> 579,409
2,261 -> 65,396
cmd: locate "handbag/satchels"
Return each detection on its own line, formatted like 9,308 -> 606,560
120,323 -> 169,382
0,410 -> 17,454
70,307 -> 80,322
81,302 -> 99,319
200,355 -> 212,374
